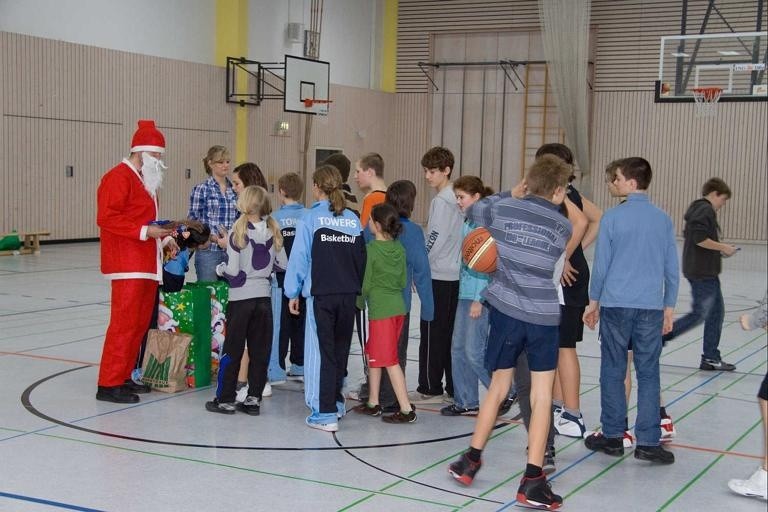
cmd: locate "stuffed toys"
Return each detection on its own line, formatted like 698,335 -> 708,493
175,223 -> 211,251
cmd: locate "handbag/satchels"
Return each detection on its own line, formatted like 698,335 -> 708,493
138,329 -> 192,393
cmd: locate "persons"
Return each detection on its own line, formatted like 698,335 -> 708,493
132,222 -> 190,381
217,162 -> 273,403
187,145 -> 240,281
97,120 -> 180,404
448,154 -> 574,512
269,144 -> 604,476
205,186 -> 288,415
664,176 -> 737,371
727,290 -> 768,500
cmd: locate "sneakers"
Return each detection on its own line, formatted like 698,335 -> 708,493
517,473 -> 562,509
206,374 -> 303,415
727,468 -> 767,500
700,355 -> 735,370
348,391 -> 479,423
543,404 -> 674,475
498,392 -> 518,416
448,453 -> 481,485
306,412 -> 341,431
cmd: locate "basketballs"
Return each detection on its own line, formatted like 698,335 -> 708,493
461,227 -> 499,274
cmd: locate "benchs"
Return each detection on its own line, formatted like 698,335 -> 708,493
1,230 -> 51,256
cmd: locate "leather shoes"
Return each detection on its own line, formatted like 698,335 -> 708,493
96,379 -> 150,403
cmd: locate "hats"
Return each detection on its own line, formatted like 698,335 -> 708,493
130,121 -> 165,153
